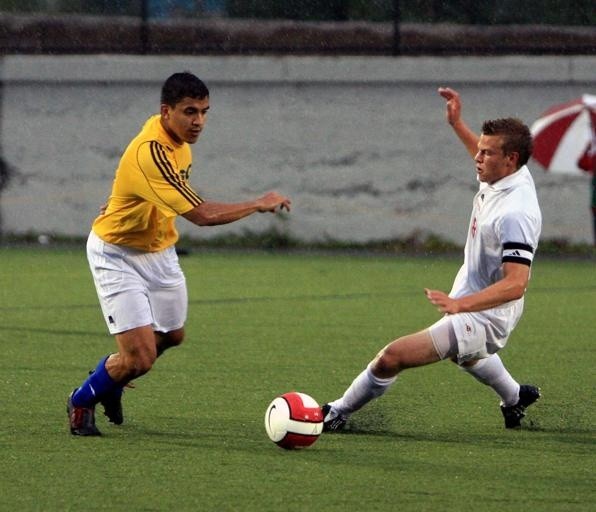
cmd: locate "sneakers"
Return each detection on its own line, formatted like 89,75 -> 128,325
65,387 -> 102,437
88,367 -> 135,426
320,401 -> 355,434
499,384 -> 542,428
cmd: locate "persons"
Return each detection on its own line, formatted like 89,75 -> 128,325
64,69 -> 292,438
318,86 -> 543,433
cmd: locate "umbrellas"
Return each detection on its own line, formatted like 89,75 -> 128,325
526,92 -> 596,176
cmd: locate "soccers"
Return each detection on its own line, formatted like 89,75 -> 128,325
264,391 -> 324,450
